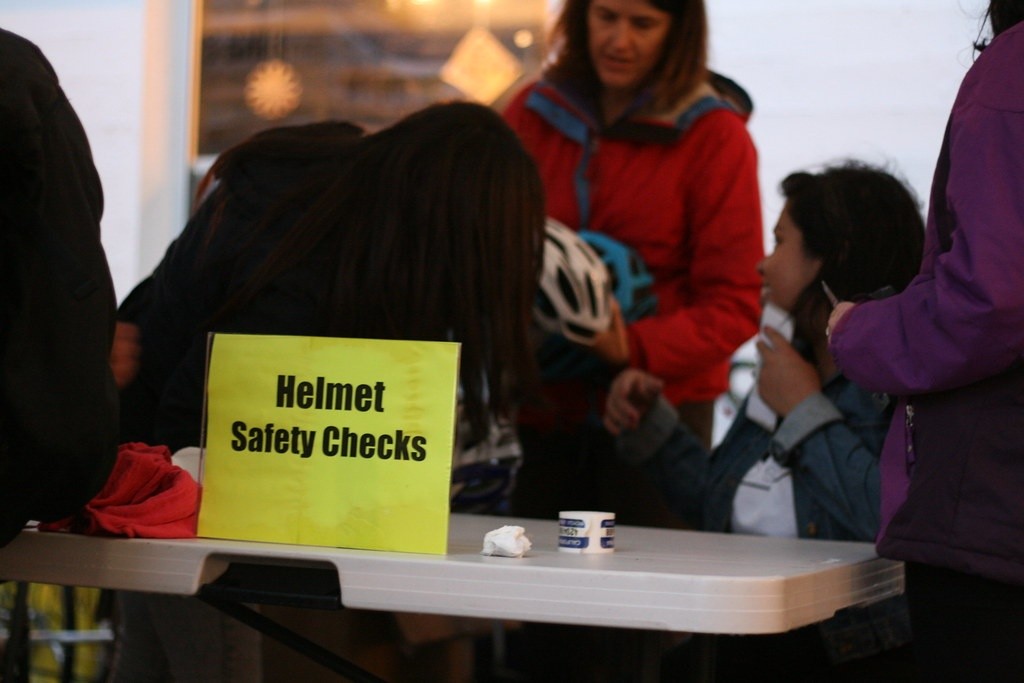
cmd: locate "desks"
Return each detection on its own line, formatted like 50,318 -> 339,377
0,514 -> 903,683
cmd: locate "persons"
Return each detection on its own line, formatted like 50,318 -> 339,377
0,28 -> 143,549
825,0 -> 1024,683
603,165 -> 924,683
106,1 -> 766,683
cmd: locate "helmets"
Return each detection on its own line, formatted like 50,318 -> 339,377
522,213 -> 614,345
568,227 -> 655,323
